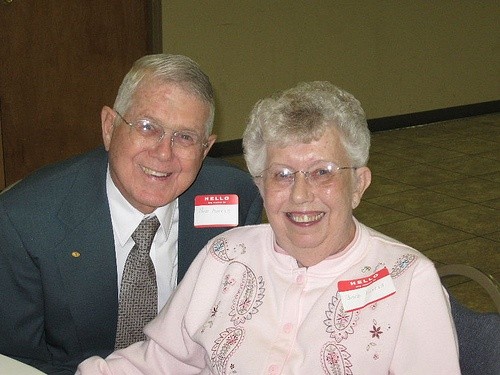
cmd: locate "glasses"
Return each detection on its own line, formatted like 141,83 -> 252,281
255,161 -> 357,193
115,110 -> 208,161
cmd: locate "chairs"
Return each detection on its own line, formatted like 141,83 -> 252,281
438,265 -> 500,375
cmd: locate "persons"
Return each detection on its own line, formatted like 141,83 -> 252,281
75,80 -> 463,375
0,54 -> 266,375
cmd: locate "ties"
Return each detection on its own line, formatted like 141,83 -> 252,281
114,216 -> 160,352
